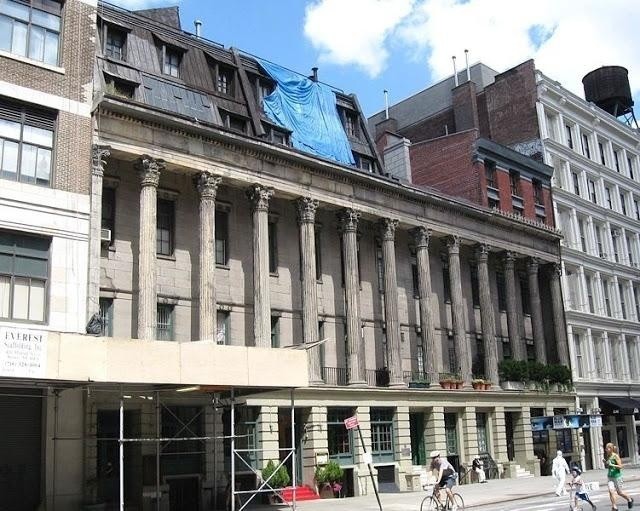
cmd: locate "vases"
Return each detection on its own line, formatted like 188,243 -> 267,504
441,380 -> 491,392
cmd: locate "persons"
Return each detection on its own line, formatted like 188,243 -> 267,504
603,443 -> 634,511
329,479 -> 348,498
568,467 -> 597,511
553,449 -> 570,496
430,450 -> 458,511
473,455 -> 487,483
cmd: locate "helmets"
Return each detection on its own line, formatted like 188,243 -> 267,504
572,466 -> 582,476
430,451 -> 441,458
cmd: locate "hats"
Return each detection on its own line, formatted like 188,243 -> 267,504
474,455 -> 480,458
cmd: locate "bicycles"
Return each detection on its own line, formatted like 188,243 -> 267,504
420,485 -> 464,510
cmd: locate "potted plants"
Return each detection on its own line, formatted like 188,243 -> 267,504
499,359 -> 572,393
262,461 -> 290,505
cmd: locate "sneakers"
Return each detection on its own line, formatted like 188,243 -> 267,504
435,504 -> 443,509
592,504 -> 597,511
556,492 -> 561,497
452,504 -> 457,511
480,480 -> 488,483
611,507 -> 619,511
628,498 -> 634,509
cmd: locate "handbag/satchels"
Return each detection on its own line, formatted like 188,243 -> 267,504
437,468 -> 454,481
